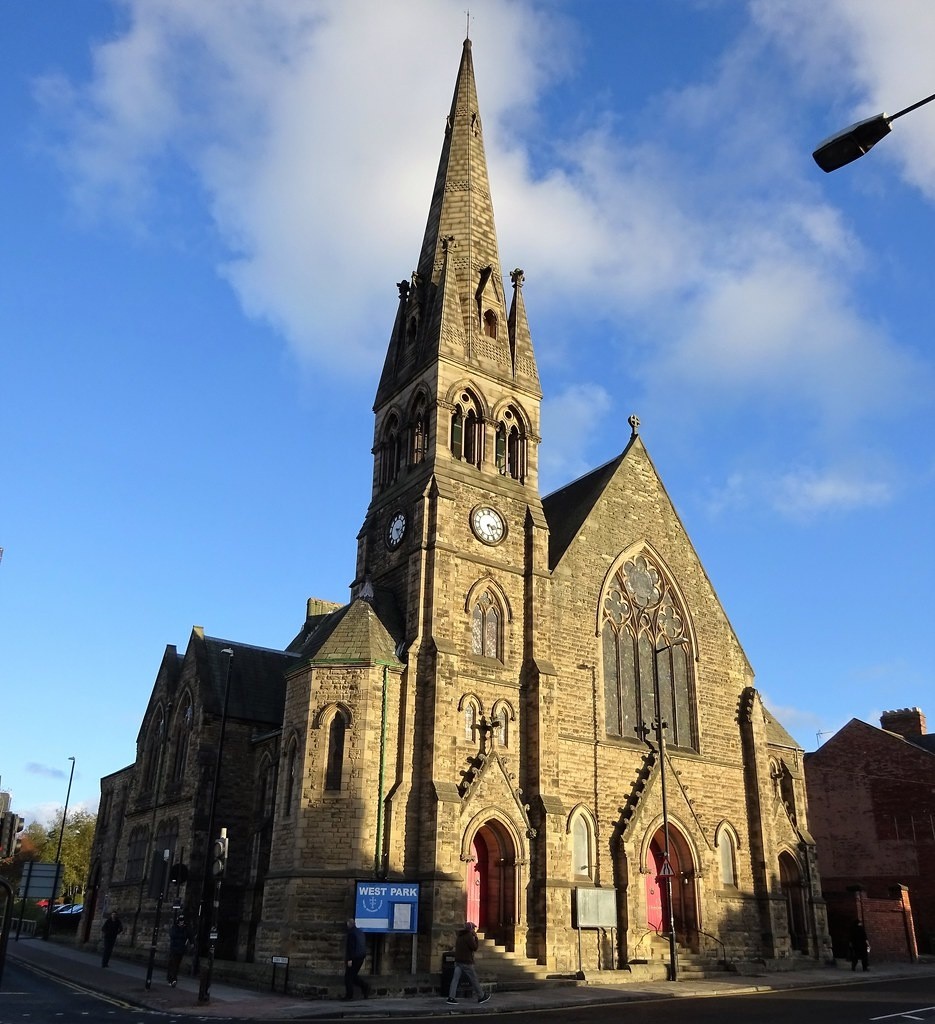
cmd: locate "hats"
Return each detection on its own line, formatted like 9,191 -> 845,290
178,915 -> 185,921
465,922 -> 478,928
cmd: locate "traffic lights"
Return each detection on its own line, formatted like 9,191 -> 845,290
0,810 -> 25,858
209,838 -> 229,880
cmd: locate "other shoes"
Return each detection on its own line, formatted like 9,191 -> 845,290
169,981 -> 177,988
341,996 -> 353,1001
862,968 -> 870,972
102,964 -> 110,969
852,968 -> 856,971
364,984 -> 371,999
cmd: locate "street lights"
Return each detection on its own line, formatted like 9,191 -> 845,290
189,647 -> 235,976
43,757 -> 76,941
651,637 -> 689,981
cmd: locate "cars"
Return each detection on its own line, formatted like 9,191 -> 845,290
47,903 -> 83,924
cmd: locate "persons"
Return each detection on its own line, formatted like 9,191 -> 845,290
101,911 -> 123,968
445,923 -> 490,1004
167,915 -> 188,987
849,919 -> 871,971
341,918 -> 376,1000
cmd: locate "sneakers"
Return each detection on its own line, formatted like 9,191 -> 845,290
478,994 -> 492,1004
446,997 -> 460,1005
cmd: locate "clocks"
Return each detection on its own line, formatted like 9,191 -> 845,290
470,503 -> 508,547
384,507 -> 409,552
